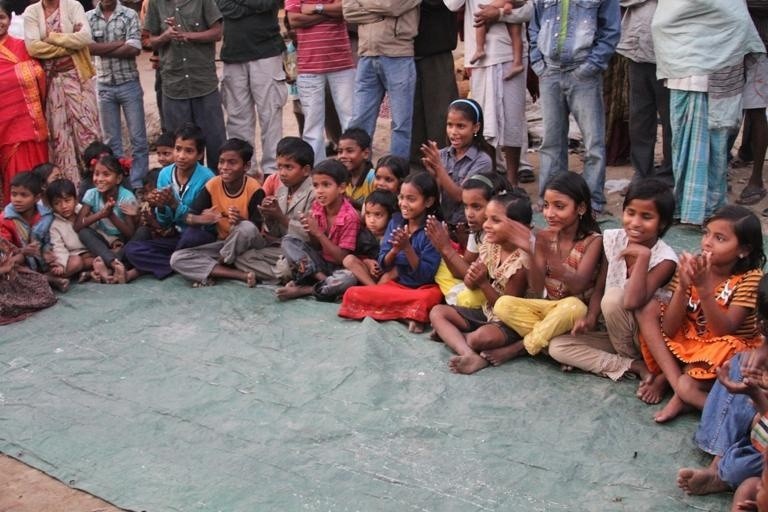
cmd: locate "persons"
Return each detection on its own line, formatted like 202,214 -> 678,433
0,0 -> 374,324
373,1 -> 767,219
339,99 -> 768,511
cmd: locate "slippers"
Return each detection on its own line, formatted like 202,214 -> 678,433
517,163 -> 535,184
735,184 -> 767,205
